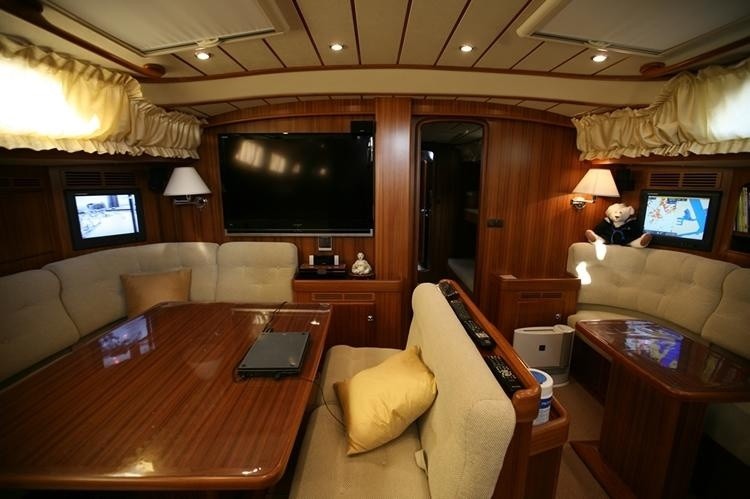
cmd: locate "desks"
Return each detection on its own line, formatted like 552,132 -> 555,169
569,318 -> 749,499
0,300 -> 334,499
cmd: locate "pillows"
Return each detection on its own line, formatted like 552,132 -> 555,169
119,267 -> 195,322
330,344 -> 438,458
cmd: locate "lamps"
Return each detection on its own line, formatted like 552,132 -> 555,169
163,166 -> 213,207
572,167 -> 621,210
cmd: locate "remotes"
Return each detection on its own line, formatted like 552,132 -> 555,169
486,355 -> 523,393
449,299 -> 472,322
463,320 -> 496,351
439,281 -> 458,300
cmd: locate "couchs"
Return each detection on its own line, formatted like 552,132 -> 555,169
0,240 -> 301,386
566,241 -> 750,468
288,281 -> 518,499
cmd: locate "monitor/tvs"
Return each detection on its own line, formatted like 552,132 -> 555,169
624,320 -> 685,370
96,312 -> 155,370
65,188 -> 147,251
216,132 -> 375,239
637,189 -> 723,252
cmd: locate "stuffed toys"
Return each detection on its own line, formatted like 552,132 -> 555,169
585,203 -> 653,249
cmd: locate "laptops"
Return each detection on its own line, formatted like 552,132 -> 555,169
238,331 -> 311,377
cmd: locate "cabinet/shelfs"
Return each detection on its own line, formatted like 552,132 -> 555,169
493,273 -> 581,349
294,280 -> 403,374
